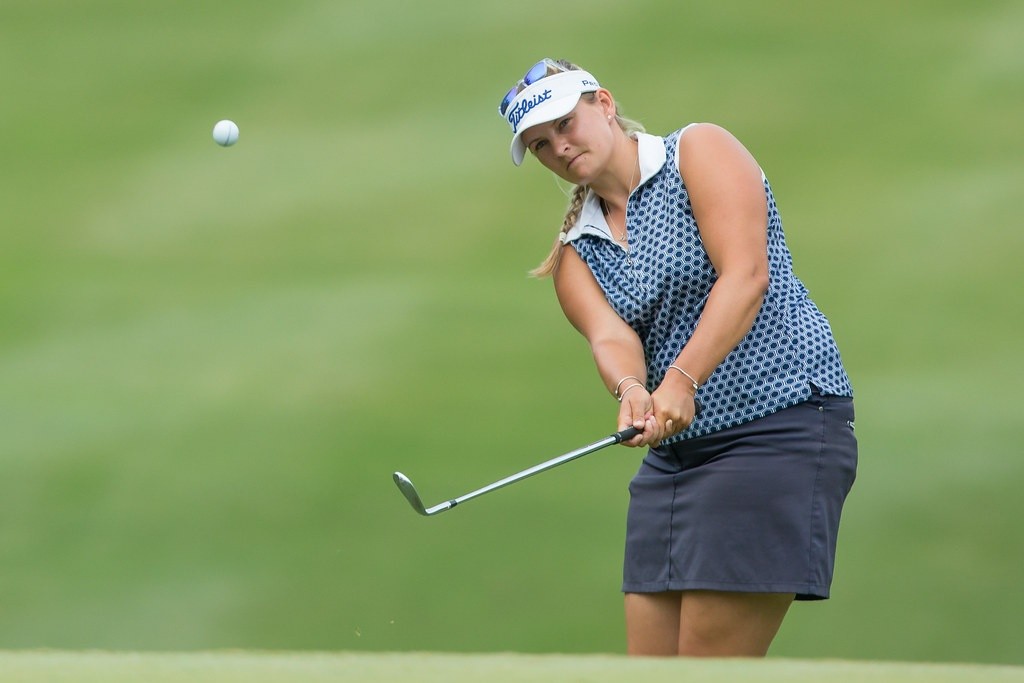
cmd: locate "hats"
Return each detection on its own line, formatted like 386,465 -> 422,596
502,69 -> 602,168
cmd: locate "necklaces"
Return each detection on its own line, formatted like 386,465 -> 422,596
603,153 -> 637,243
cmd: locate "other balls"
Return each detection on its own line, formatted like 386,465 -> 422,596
212,120 -> 240,148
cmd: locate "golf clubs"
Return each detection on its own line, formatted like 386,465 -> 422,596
391,397 -> 703,519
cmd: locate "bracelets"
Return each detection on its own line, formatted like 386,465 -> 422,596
614,376 -> 646,402
667,365 -> 699,392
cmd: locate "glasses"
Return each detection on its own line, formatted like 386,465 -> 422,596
500,57 -> 570,115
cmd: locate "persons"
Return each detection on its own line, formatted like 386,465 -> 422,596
498,58 -> 858,658
